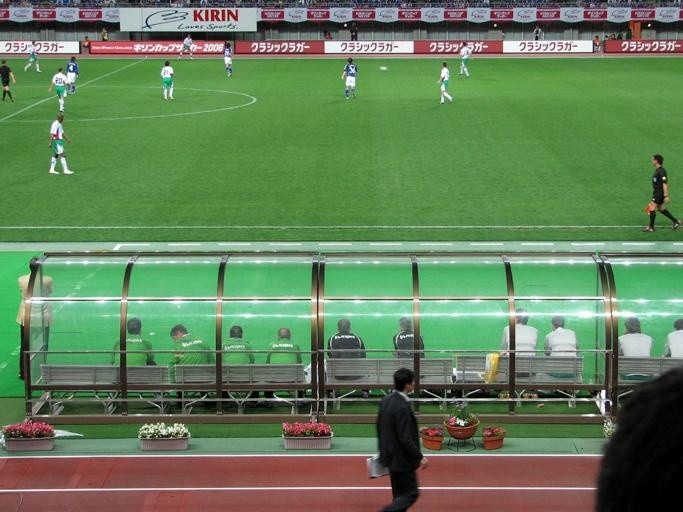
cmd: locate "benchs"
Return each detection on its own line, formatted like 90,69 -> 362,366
173,364 -> 309,414
38,363 -> 170,415
617,357 -> 682,408
323,357 -> 450,411
456,355 -> 583,409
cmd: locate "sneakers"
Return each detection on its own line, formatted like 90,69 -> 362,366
672,220 -> 680,230
640,226 -> 655,232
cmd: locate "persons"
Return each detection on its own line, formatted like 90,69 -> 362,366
457,41 -> 472,79
593,28 -> 634,52
46,67 -> 68,113
110,317 -> 157,366
265,329 -> 306,408
81,35 -> 92,48
47,113 -> 75,176
498,307 -> 538,357
177,32 -> 194,60
348,20 -> 358,41
0,58 -> 17,103
531,25 -> 542,41
13,255 -> 53,380
340,57 -> 360,100
640,152 -> 682,232
327,319 -> 370,398
618,317 -> 654,381
221,325 -> 259,406
544,316 -> 582,396
23,40 -> 42,74
666,318 -> 683,359
67,56 -> 79,95
588,366 -> 681,512
323,29 -> 333,39
101,26 -> 109,41
435,61 -> 453,106
374,364 -> 428,511
168,324 -> 217,399
393,317 -> 425,359
159,59 -> 176,103
222,43 -> 233,79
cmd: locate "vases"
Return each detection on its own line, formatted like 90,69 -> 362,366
444,418 -> 480,439
139,435 -> 191,450
421,433 -> 443,450
5,436 -> 58,450
483,436 -> 504,450
282,432 -> 333,449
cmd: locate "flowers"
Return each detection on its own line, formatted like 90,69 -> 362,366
2,418 -> 56,439
603,421 -> 621,439
420,424 -> 444,437
137,421 -> 192,439
482,425 -> 506,438
280,421 -> 333,438
446,409 -> 478,427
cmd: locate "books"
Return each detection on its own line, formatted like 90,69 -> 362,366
364,453 -> 390,480
